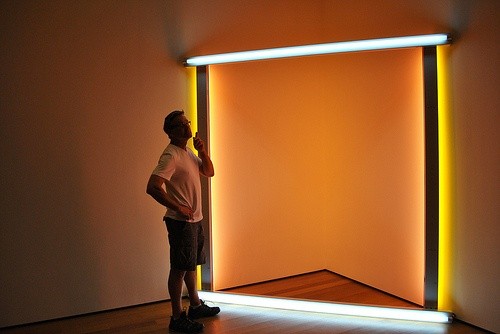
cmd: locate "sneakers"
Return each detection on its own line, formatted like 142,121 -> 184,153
188,299 -> 221,319
168,311 -> 205,333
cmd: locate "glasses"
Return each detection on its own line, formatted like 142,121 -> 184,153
172,121 -> 192,128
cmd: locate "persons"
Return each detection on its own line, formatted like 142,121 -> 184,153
146,110 -> 220,332
